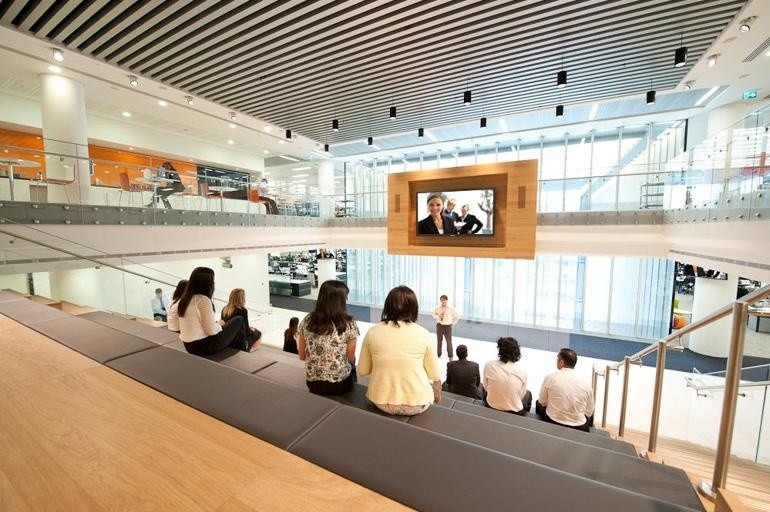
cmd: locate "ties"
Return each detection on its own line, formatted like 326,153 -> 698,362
416,186 -> 496,237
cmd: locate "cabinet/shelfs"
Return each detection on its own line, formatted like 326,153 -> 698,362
267,247 -> 346,297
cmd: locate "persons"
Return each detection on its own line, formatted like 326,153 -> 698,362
482,335 -> 533,418
355,285 -> 443,416
149,266 -> 262,356
535,348 -> 596,433
431,295 -> 460,364
143,162 -> 184,209
443,343 -> 483,400
283,316 -> 299,354
417,192 -> 483,236
298,281 -> 356,395
255,178 -> 279,215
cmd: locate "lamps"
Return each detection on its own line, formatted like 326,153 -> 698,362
480,107 -> 487,128
229,112 -> 236,120
556,94 -> 564,117
708,54 -> 717,67
647,77 -> 656,105
670,262 -> 770,346
739,16 -> 754,34
464,78 -> 471,105
684,80 -> 694,90
418,122 -> 424,137
286,123 -> 291,141
674,30 -> 687,68
185,95 -> 194,106
129,76 -> 139,88
557,58 -> 567,88
390,96 -> 396,120
50,44 -> 63,61
333,111 -> 339,131
325,137 -> 329,152
368,128 -> 373,146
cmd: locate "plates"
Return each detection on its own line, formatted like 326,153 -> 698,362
267,247 -> 346,297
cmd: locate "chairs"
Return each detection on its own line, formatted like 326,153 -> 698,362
670,262 -> 770,346
1,154 -> 357,219
